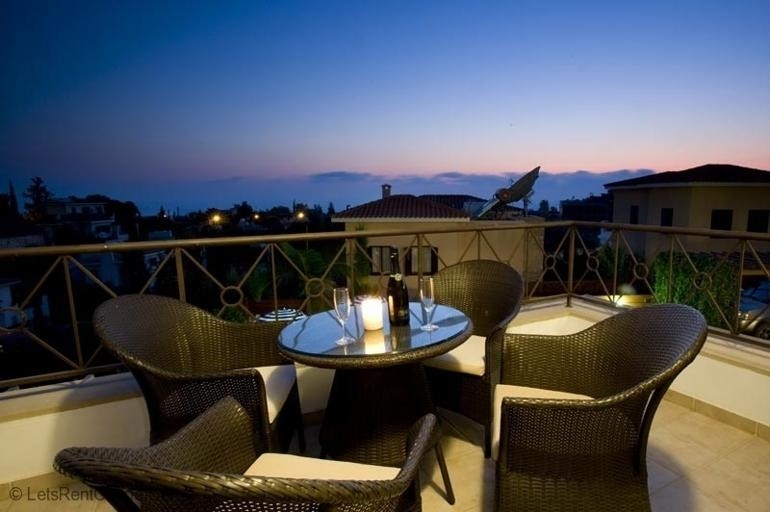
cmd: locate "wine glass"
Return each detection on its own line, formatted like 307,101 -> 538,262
332,287 -> 356,346
417,275 -> 441,333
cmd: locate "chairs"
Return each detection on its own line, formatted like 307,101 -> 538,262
489,302 -> 708,512
422,258 -> 524,459
91,293 -> 305,454
53,395 -> 435,512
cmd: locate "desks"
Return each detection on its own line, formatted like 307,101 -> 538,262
276,302 -> 473,505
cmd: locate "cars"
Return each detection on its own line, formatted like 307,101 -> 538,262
738,282 -> 770,338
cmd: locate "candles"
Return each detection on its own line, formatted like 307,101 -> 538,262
360,295 -> 383,330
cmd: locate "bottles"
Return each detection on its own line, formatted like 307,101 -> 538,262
384,245 -> 411,327
